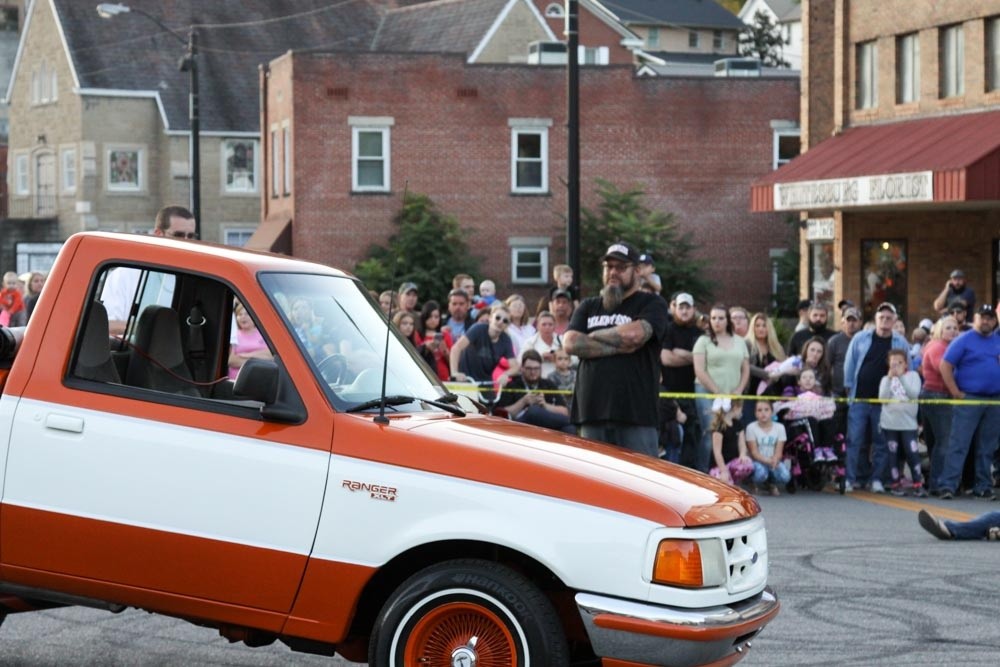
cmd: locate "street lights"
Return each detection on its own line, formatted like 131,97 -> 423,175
95,2 -> 202,239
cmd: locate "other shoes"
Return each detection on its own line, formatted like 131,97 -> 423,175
748,446 -> 997,501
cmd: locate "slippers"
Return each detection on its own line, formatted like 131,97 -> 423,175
918,509 -> 952,540
986,526 -> 1000,540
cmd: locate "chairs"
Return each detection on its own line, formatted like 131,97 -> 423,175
73,299 -> 121,384
130,305 -> 201,397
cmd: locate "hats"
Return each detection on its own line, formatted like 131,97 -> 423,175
551,288 -> 571,301
676,293 -> 694,308
973,303 -> 997,317
839,300 -> 854,308
603,243 -> 640,266
950,269 -> 967,278
876,302 -> 897,314
398,282 -> 418,296
637,254 -> 655,265
845,307 -> 863,321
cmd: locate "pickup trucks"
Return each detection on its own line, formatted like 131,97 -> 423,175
0,231 -> 780,667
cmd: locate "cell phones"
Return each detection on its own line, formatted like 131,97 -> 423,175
433,333 -> 444,342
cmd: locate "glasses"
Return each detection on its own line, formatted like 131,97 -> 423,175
164,228 -> 198,240
809,303 -> 826,309
878,315 -> 891,321
949,310 -> 964,315
491,315 -> 509,324
524,366 -> 542,371
603,263 -> 634,273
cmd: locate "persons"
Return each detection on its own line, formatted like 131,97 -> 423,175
562,241 -> 667,459
918,508 -> 1000,541
636,254 -> 1000,500
228,263 -> 580,435
0,271 -> 49,327
99,207 -> 196,344
